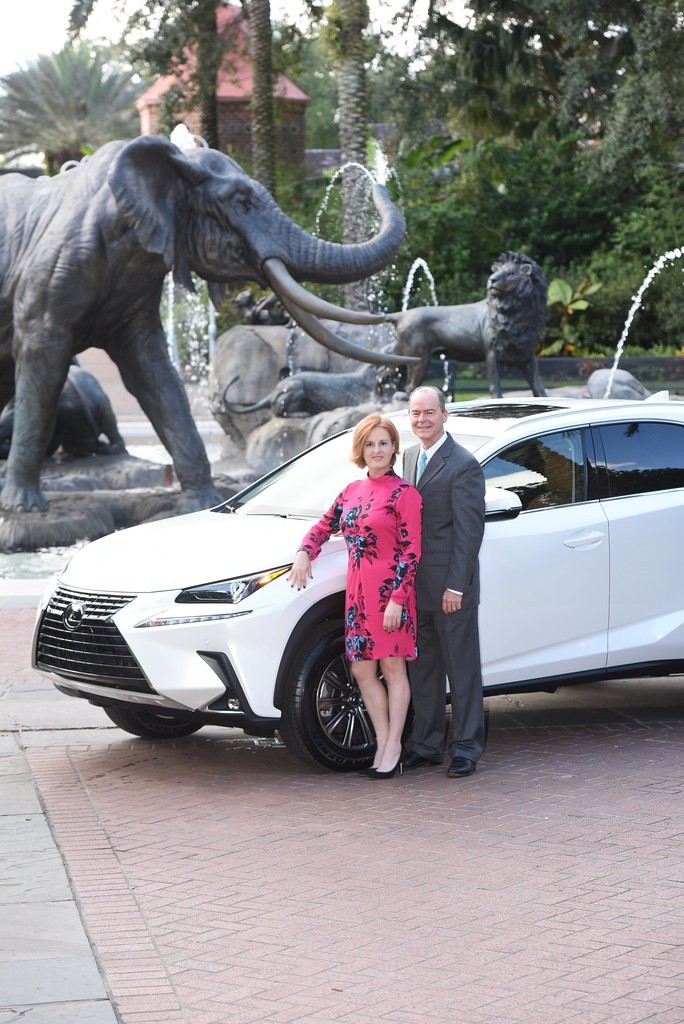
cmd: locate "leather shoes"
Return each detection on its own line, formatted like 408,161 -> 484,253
401,750 -> 476,778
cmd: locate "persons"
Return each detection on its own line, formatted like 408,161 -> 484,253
402,385 -> 487,778
288,414 -> 423,780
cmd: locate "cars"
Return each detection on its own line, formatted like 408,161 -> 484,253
31,387 -> 684,772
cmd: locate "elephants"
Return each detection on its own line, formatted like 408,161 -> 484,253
1,123 -> 423,520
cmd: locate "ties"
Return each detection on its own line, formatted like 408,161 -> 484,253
416,451 -> 427,488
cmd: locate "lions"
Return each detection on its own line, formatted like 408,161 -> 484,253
385,250 -> 549,400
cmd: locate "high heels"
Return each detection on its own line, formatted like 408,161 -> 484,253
355,741 -> 407,779
